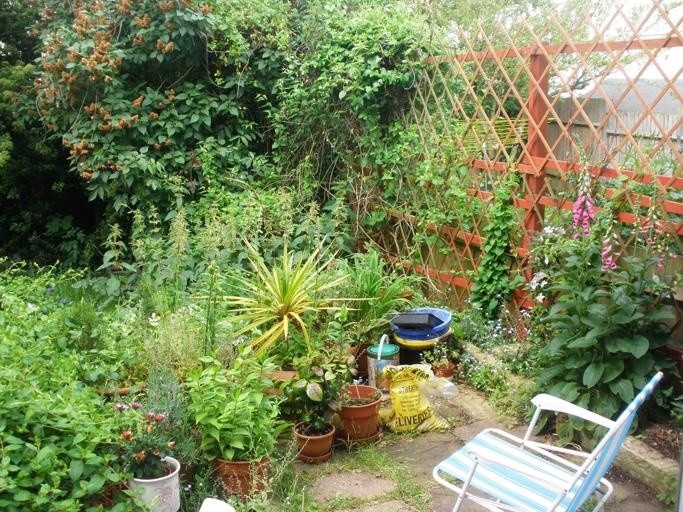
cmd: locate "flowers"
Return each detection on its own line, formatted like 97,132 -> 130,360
108,403 -> 176,478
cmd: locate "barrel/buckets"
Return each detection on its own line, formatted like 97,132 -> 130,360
367,336 -> 401,389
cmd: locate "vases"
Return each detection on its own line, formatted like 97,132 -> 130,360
191,345 -> 382,501
127,459 -> 181,512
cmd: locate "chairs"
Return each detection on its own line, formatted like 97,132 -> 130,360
432,371 -> 665,512
